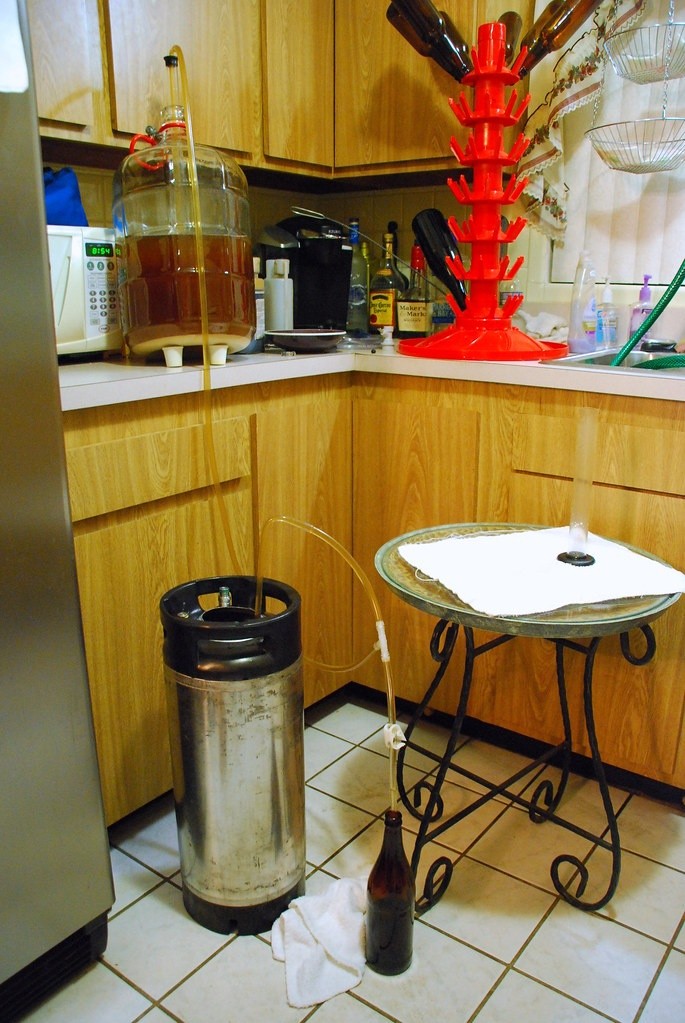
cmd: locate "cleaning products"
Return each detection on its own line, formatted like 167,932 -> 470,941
595,276 -> 618,351
568,250 -> 599,353
627,274 -> 659,351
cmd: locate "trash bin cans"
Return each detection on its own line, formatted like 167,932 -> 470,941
160,576 -> 305,935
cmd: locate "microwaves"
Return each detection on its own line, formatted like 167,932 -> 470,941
46,225 -> 123,355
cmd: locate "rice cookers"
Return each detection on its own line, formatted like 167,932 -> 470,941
253,217 -> 355,330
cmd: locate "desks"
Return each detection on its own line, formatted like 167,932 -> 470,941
374,525 -> 682,912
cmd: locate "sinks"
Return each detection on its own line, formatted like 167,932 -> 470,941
575,351 -> 681,370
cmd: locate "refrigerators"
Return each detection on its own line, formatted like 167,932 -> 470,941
0,2 -> 117,1023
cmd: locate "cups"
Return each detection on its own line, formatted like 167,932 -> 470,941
162,346 -> 184,367
209,344 -> 229,365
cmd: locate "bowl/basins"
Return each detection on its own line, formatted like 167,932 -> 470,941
267,328 -> 347,350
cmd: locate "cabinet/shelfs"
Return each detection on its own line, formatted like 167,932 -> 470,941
24,0 -> 541,183
68,370 -> 684,828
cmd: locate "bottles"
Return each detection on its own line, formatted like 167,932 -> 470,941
499,267 -> 523,305
364,810 -> 415,976
385,0 -> 603,83
346,208 -> 467,339
111,104 -> 257,357
252,255 -> 294,350
